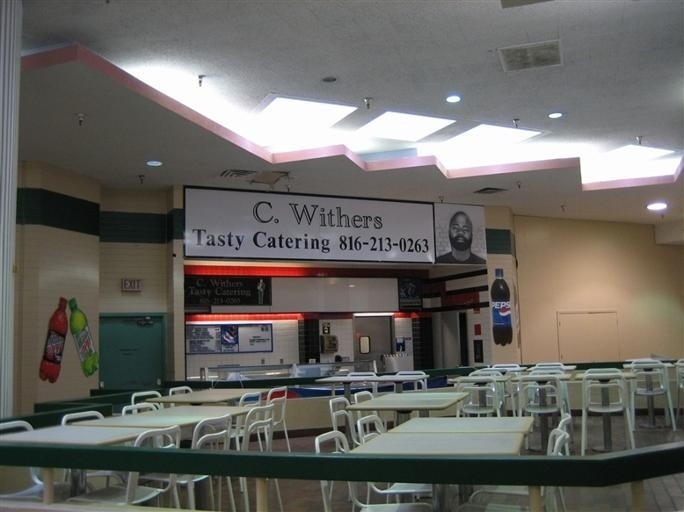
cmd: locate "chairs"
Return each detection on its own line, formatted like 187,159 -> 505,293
0,362 -> 684,512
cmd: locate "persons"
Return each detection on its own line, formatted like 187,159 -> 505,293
436,211 -> 486,264
257,279 -> 265,305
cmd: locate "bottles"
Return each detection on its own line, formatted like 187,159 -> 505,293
40,297 -> 100,383
491,268 -> 513,346
